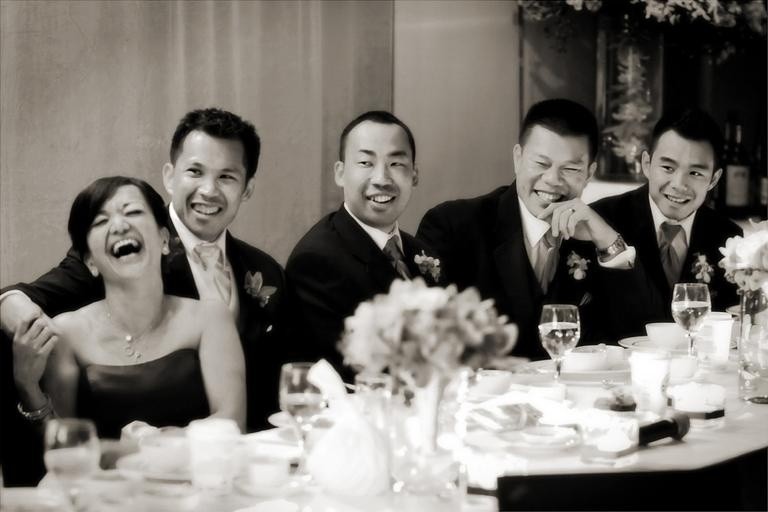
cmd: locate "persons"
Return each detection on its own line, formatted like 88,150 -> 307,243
284,111 -> 455,395
416,97 -> 660,360
0,108 -> 290,436
10,176 -> 246,470
586,112 -> 743,312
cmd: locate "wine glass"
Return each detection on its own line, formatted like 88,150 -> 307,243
42,414 -> 102,508
672,283 -> 712,354
539,303 -> 582,380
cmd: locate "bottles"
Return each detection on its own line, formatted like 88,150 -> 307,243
720,123 -> 767,221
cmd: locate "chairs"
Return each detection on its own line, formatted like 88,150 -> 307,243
496,445 -> 766,510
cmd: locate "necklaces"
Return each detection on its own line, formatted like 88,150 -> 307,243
104,302 -> 165,358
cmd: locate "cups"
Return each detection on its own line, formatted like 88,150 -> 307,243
114,351 -> 675,512
700,311 -> 733,370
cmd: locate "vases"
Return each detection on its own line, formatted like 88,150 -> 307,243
405,377 -> 448,457
738,289 -> 768,404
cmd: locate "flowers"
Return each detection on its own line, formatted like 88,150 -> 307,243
718,218 -> 768,325
339,279 -> 522,387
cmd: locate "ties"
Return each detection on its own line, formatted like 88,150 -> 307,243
538,229 -> 565,294
205,244 -> 233,313
658,222 -> 682,293
383,237 -> 414,285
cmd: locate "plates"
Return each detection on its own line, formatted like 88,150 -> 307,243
726,304 -> 741,315
617,335 -> 688,353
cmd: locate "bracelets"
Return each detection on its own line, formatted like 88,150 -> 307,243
594,233 -> 627,259
17,392 -> 54,421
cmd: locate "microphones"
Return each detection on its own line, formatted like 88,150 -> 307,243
629,412 -> 690,444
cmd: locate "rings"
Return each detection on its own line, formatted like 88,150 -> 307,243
568,208 -> 575,212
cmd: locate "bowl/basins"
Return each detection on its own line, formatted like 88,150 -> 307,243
567,344 -> 624,369
673,356 -> 698,378
644,321 -> 690,348
